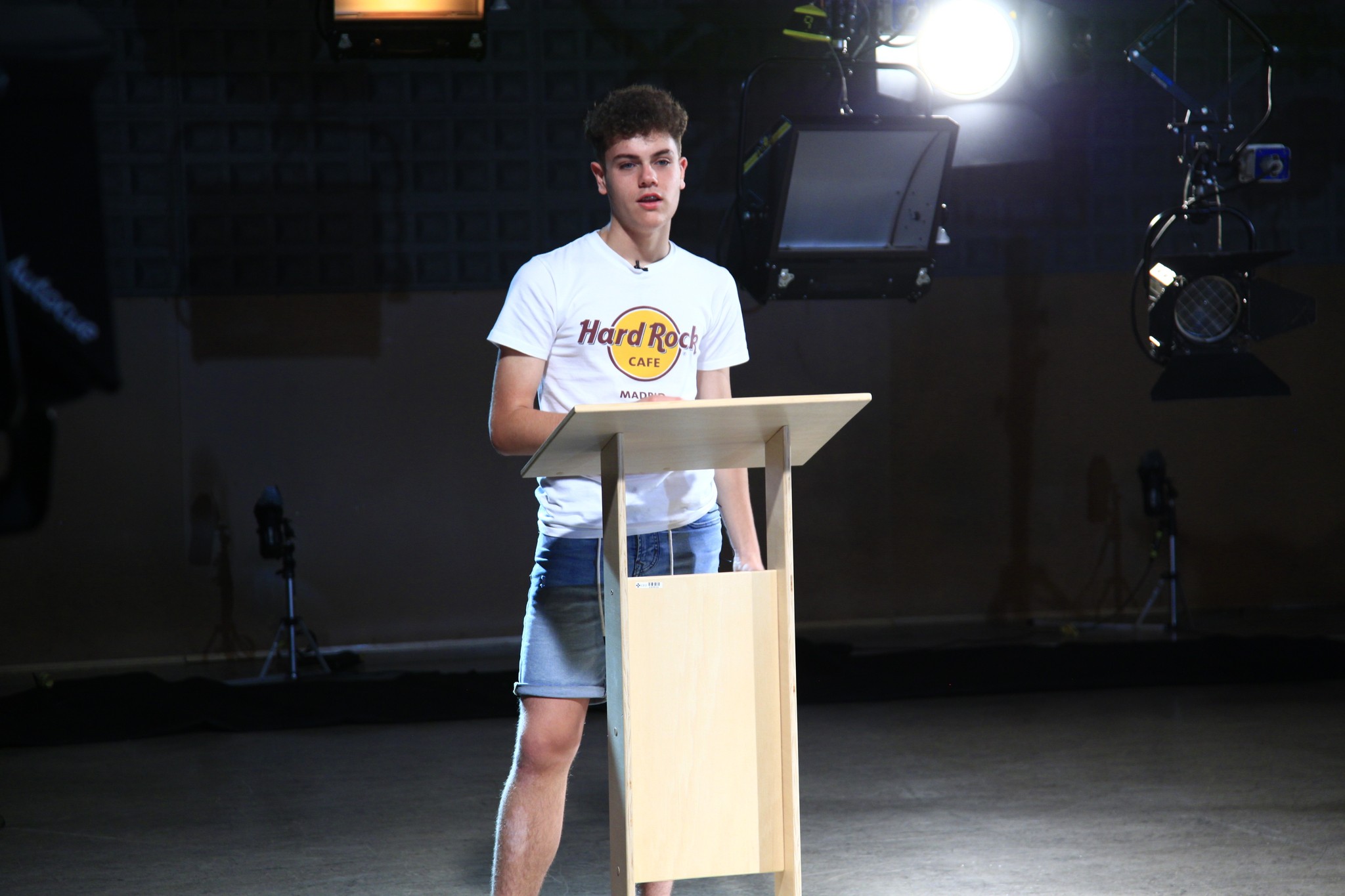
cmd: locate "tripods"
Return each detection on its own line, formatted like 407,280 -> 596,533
1138,492 -> 1193,639
254,538 -> 331,681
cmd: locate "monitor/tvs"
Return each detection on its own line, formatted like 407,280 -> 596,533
765,113 -> 960,298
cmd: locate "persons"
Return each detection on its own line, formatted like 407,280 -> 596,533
484,85 -> 765,896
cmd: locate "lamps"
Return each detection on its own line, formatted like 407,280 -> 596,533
1139,245 -> 1316,404
